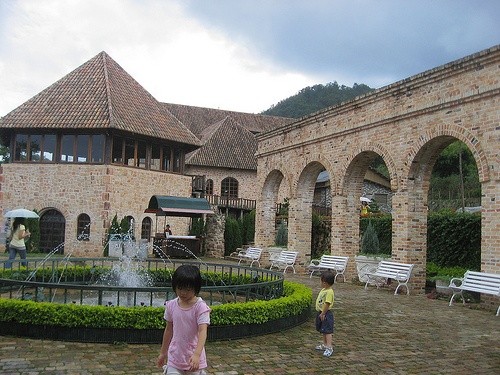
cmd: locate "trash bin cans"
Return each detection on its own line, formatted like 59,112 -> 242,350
139,239 -> 149,259
124,234 -> 137,258
109,234 -> 121,257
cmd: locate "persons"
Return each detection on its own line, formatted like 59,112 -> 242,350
3,217 -> 31,269
360,201 -> 371,217
316,270 -> 334,358
164,224 -> 172,235
160,265 -> 211,375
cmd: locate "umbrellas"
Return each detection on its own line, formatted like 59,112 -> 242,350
360,197 -> 371,203
4,208 -> 39,237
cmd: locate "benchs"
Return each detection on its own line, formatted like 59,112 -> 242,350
268,251 -> 299,275
308,254 -> 350,282
362,261 -> 415,295
449,271 -> 500,317
237,248 -> 262,269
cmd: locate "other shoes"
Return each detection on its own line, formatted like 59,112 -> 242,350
323,347 -> 334,357
315,344 -> 328,350
